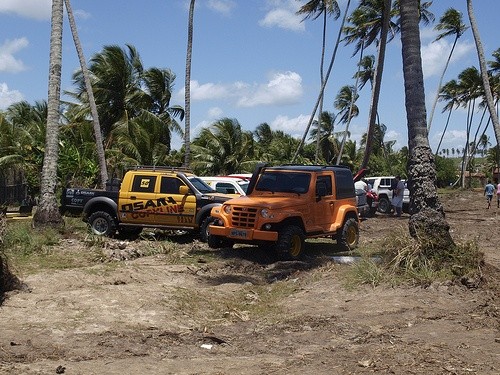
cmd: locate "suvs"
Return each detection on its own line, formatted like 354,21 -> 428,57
205,165 -> 360,257
363,177 -> 412,214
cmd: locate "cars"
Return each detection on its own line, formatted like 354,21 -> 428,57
188,172 -> 253,194
352,168 -> 381,216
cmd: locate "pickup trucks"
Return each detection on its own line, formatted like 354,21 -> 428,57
65,166 -> 242,238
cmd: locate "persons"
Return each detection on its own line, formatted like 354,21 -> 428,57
496,184 -> 500,208
355,178 -> 373,213
484,180 -> 495,209
388,175 -> 404,217
